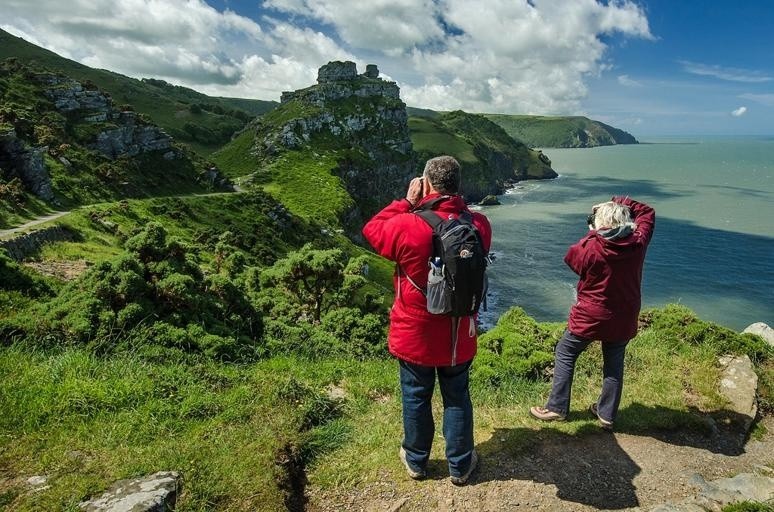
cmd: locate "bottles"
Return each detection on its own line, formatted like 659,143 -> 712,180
433,257 -> 442,278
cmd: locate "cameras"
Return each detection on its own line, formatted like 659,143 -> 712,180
587,207 -> 600,228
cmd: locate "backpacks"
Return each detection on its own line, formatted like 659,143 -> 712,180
395,210 -> 489,368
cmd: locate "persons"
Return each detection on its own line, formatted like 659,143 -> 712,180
529,192 -> 657,432
361,155 -> 492,485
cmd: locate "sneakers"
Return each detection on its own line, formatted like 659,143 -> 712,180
399,446 -> 426,479
529,407 -> 567,421
450,449 -> 478,485
590,403 -> 614,429
